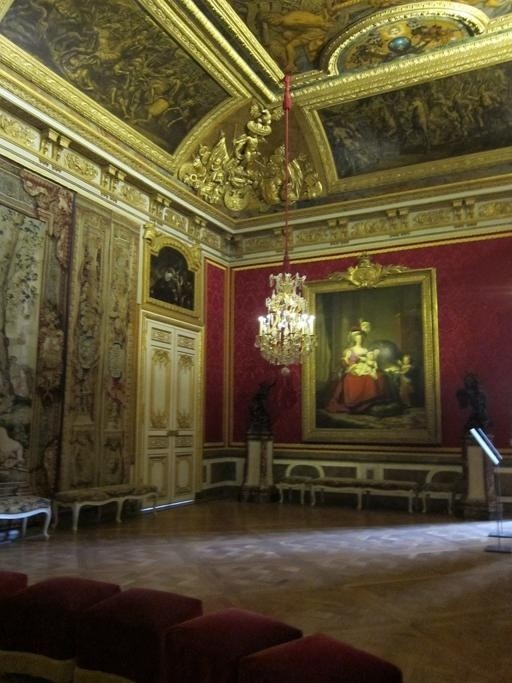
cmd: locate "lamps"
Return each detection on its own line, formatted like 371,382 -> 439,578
254,76 -> 320,376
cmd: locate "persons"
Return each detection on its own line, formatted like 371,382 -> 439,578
343,348 -> 381,379
148,258 -> 195,310
2,0 -> 234,165
453,372 -> 492,435
392,353 -> 415,409
313,57 -> 510,181
343,325 -> 381,406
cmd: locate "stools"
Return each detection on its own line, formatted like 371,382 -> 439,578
0,483 -> 160,538
274,476 -> 457,516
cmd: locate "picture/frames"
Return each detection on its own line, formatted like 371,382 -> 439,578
143,222 -> 201,326
298,256 -> 443,446
